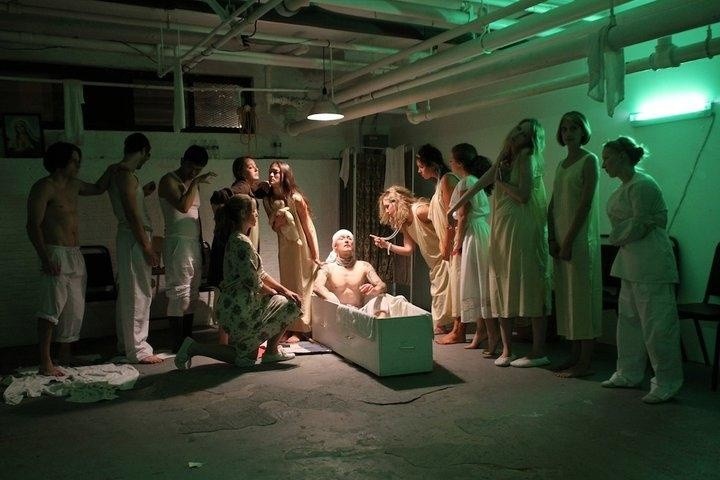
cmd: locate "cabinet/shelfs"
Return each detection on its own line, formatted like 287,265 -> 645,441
310,295 -> 434,379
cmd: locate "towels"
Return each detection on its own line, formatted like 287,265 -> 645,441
172,56 -> 188,133
587,25 -> 625,117
339,144 -> 350,189
384,143 -> 407,189
63,79 -> 85,137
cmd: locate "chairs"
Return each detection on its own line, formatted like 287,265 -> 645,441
80,243 -> 117,303
198,239 -> 214,292
601,233 -> 681,376
677,242 -> 720,391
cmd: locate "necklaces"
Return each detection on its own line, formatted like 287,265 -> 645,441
369,224 -> 402,240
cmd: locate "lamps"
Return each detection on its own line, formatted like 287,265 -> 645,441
306,38 -> 344,122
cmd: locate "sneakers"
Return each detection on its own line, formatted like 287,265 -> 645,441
494,355 -> 513,366
262,351 -> 295,365
509,357 -> 550,367
642,394 -> 661,403
175,337 -> 194,370
601,381 -> 616,388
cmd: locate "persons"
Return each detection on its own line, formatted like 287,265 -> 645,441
547,111 -> 604,379
448,143 -> 500,358
374,185 -> 454,335
174,194 -> 304,373
414,143 -> 467,344
158,145 -> 218,348
602,137 -> 685,404
263,161 -> 327,343
313,229 -> 390,318
26,141 -> 110,378
107,132 -> 164,364
446,118 -> 551,368
205,156 -> 271,346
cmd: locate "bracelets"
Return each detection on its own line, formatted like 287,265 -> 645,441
387,243 -> 392,255
548,238 -> 556,243
